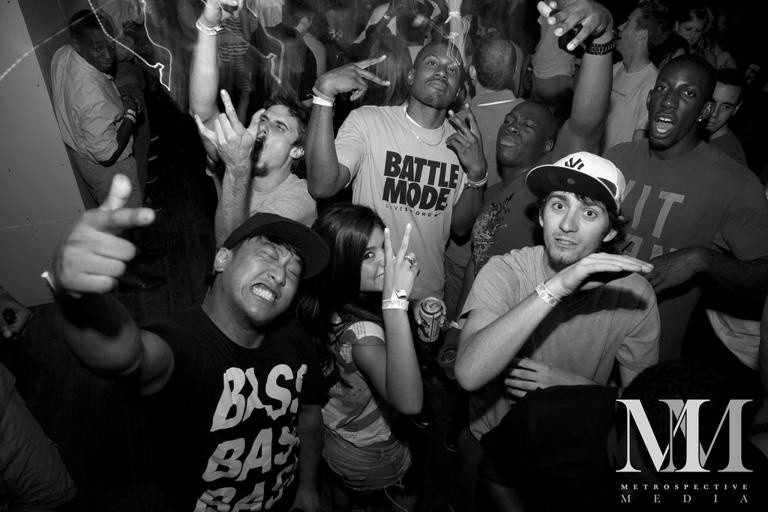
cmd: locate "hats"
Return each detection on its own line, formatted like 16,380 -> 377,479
525,152 -> 626,216
224,213 -> 330,281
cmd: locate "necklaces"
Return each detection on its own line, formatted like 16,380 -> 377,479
403,106 -> 445,146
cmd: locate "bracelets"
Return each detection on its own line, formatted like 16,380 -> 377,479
381,290 -> 409,310
535,282 -> 561,309
124,114 -> 137,123
196,19 -> 224,35
312,96 -> 334,107
312,87 -> 335,103
124,109 -> 136,116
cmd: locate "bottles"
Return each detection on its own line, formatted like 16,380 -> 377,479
417,297 -> 443,344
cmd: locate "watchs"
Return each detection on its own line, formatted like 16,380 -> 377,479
586,29 -> 619,56
465,171 -> 489,189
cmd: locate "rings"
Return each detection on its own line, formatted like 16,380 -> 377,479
404,255 -> 416,264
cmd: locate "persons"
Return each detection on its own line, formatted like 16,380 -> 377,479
50,173 -> 326,512
335,39 -> 413,107
603,5 -> 662,155
603,53 -> 768,363
304,39 -> 489,432
328,2 -> 536,38
703,67 -> 748,166
439,1 -> 616,395
191,0 -> 316,248
216,0 -> 260,126
50,11 -> 144,209
478,363 -> 768,511
652,32 -> 692,71
671,6 -> 717,65
0,283 -> 79,512
247,1 -> 318,124
442,37 -> 526,354
456,151 -> 661,512
312,203 -> 447,512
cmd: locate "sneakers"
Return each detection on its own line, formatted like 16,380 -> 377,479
440,418 -> 464,457
411,400 -> 435,429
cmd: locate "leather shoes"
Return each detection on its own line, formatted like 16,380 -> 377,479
119,276 -> 168,291
